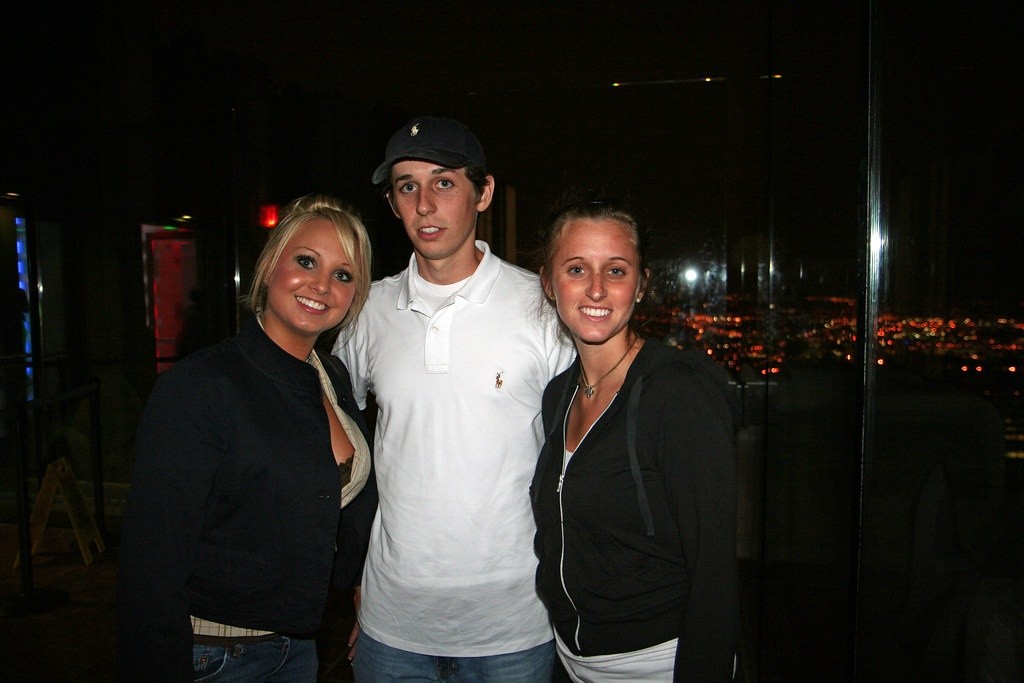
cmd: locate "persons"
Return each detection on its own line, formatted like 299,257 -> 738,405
327,118 -> 577,683
530,199 -> 740,683
126,193 -> 373,683
177,287 -> 208,360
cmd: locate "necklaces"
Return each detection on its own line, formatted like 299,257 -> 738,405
579,334 -> 639,399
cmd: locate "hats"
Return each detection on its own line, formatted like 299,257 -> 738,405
371,117 -> 485,185
